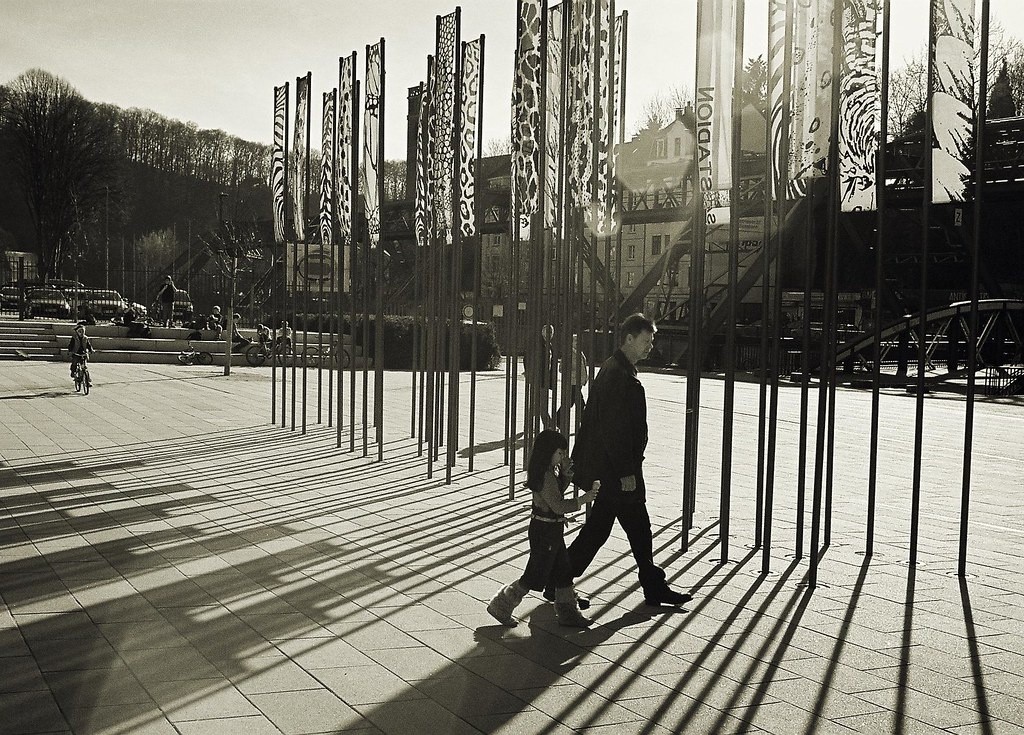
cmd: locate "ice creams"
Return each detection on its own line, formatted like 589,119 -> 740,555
592,480 -> 601,491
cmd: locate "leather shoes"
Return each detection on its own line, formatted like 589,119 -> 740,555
543,582 -> 589,610
644,582 -> 691,607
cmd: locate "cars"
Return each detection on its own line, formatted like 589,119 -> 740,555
156,289 -> 194,323
1,279 -> 148,319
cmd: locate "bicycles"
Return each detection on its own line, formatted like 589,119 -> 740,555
246,337 -> 297,367
177,339 -> 214,366
69,351 -> 96,395
299,334 -> 351,368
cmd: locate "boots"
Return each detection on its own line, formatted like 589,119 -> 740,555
554,585 -> 594,627
487,580 -> 527,627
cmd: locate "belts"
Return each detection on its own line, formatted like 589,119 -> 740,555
531,514 -> 566,522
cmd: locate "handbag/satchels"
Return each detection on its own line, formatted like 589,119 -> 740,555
186,332 -> 201,340
570,440 -> 602,491
196,315 -> 206,329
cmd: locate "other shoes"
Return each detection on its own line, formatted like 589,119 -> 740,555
86,382 -> 92,387
216,336 -> 222,341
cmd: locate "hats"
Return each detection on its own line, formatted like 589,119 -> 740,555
76,326 -> 85,332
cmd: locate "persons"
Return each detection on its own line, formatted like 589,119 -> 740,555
553,334 -> 589,429
69,324 -> 95,387
487,429 -> 599,629
543,312 -> 692,609
521,326 -> 556,430
114,275 -> 293,355
